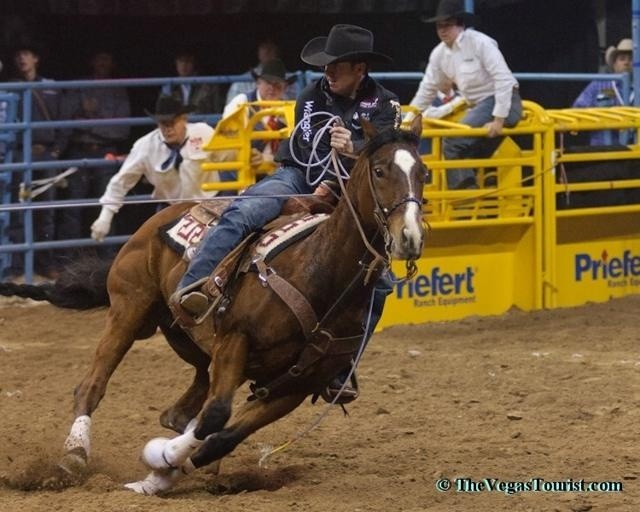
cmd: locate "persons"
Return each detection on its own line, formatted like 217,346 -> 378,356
169,23 -> 403,400
0,2 -> 639,277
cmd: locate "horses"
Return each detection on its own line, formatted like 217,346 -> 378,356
48,112 -> 426,498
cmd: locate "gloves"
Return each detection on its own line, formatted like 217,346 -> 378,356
89,205 -> 114,245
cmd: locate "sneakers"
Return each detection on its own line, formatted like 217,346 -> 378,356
179,291 -> 209,319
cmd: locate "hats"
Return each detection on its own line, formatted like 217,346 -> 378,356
420,1 -> 476,25
251,57 -> 298,86
605,38 -> 633,66
143,93 -> 199,122
299,23 -> 393,68
